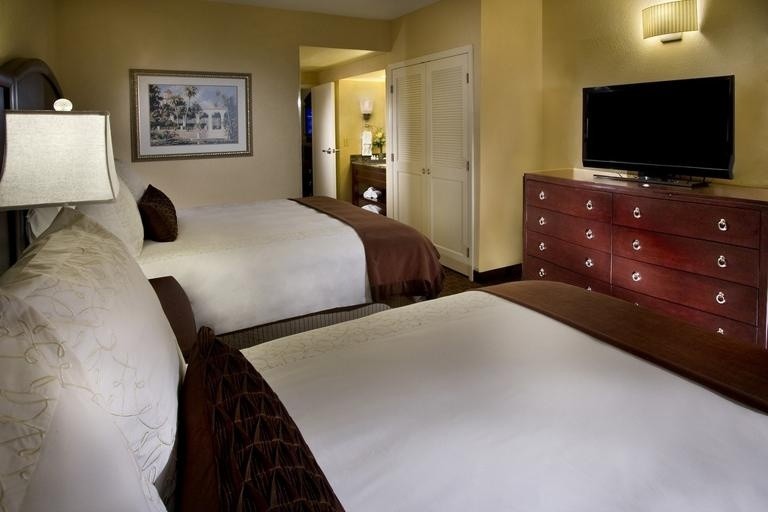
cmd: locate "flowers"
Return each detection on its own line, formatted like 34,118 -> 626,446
372,126 -> 385,147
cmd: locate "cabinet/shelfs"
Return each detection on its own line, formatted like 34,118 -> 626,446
351,161 -> 386,215
522,169 -> 609,299
609,191 -> 766,346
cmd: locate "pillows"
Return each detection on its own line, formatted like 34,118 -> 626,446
23,202 -> 73,242
0,295 -> 165,511
166,322 -> 350,509
136,183 -> 178,243
71,177 -> 145,262
1,208 -> 186,485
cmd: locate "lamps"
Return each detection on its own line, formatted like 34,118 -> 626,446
0,109 -> 121,262
640,0 -> 701,45
358,95 -> 374,121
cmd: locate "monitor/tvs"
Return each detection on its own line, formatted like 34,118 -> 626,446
581,74 -> 736,187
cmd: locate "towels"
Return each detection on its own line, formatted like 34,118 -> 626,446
364,185 -> 381,200
360,203 -> 384,214
361,130 -> 374,156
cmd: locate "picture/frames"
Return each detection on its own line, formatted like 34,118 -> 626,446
127,66 -> 254,162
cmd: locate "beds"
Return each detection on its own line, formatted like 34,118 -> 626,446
0,192 -> 445,351
0,278 -> 767,510
